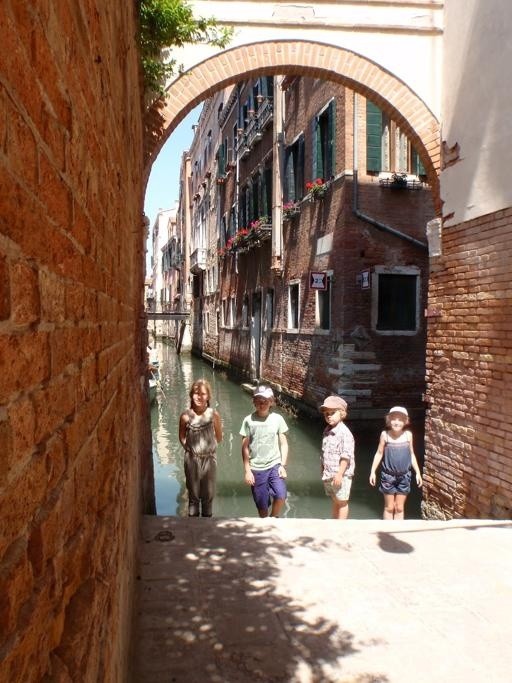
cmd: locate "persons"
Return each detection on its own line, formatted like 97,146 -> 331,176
147,345 -> 160,379
177,376 -> 224,516
316,393 -> 357,518
368,405 -> 425,520
237,382 -> 291,515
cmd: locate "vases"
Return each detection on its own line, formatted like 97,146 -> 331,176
287,210 -> 295,218
314,188 -> 324,198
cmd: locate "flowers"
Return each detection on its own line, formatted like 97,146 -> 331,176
217,215 -> 268,257
283,200 -> 294,213
306,178 -> 327,193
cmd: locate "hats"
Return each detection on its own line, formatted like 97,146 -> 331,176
388,405 -> 408,417
318,394 -> 348,413
251,383 -> 275,399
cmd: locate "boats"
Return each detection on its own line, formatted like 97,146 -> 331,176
147,346 -> 161,404
